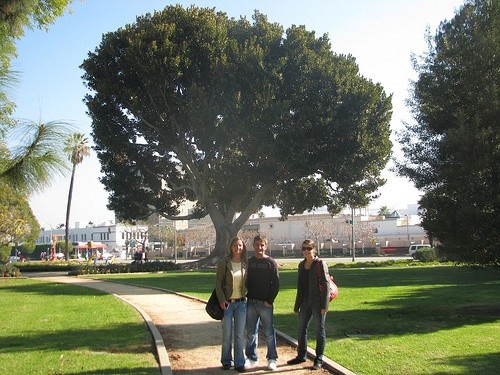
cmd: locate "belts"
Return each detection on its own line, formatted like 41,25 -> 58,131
228,298 -> 246,303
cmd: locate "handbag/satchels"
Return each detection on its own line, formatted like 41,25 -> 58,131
317,259 -> 339,304
206,289 -> 228,320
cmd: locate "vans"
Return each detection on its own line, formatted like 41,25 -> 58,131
409,244 -> 434,260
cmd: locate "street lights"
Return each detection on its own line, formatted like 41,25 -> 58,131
405,214 -> 409,233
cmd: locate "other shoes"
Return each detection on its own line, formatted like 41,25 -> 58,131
268,360 -> 277,369
288,359 -> 306,365
235,367 -> 246,372
244,359 -> 257,367
223,364 -> 231,369
313,364 -> 322,370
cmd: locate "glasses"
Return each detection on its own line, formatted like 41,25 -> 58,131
303,246 -> 314,251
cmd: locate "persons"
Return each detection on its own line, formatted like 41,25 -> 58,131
215,236 -> 250,373
244,235 -> 280,369
134,250 -> 148,261
286,239 -> 330,369
93,251 -> 97,264
